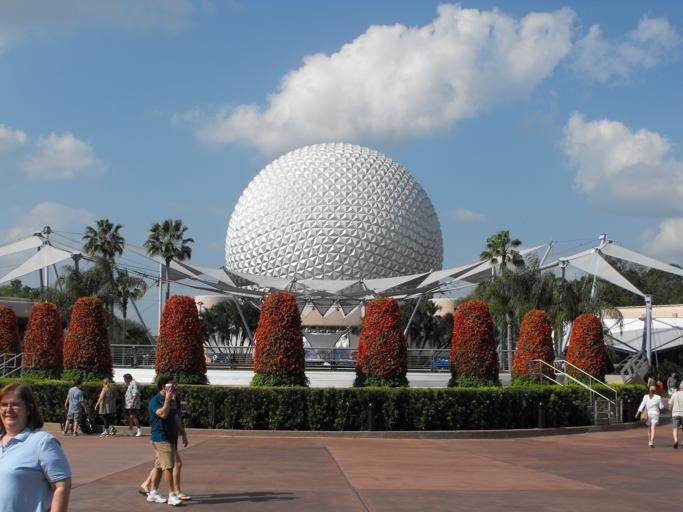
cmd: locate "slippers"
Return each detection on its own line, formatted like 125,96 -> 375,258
175,492 -> 191,501
137,484 -> 150,497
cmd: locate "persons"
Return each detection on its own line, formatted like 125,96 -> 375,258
145,377 -> 188,504
70,406 -> 93,434
61,379 -> 88,435
137,384 -> 191,500
121,373 -> 143,437
646,374 -> 655,387
655,377 -> 663,394
667,381 -> 683,447
634,385 -> 667,447
0,381 -> 72,511
667,371 -> 678,395
93,378 -> 117,438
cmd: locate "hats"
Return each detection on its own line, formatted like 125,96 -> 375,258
650,386 -> 656,391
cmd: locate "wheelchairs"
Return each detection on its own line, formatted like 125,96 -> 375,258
59,403 -> 95,433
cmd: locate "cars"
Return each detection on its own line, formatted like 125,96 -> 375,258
320,350 -> 349,360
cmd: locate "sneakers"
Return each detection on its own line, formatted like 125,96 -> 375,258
109,426 -> 118,436
673,440 -> 679,449
97,431 -> 109,438
647,441 -> 656,448
134,428 -> 145,436
122,429 -> 134,436
166,491 -> 182,506
145,489 -> 167,505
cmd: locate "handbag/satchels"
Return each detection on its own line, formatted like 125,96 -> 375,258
639,406 -> 648,423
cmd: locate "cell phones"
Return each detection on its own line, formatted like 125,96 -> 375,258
166,384 -> 172,392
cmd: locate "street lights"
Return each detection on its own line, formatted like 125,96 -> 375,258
196,301 -> 203,318
